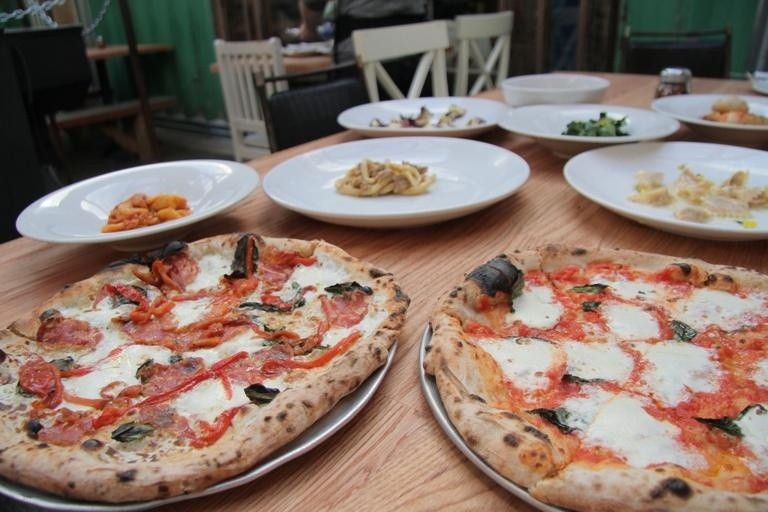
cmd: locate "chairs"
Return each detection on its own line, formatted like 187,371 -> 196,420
214,38 -> 302,156
250,58 -> 373,149
456,9 -> 512,95
350,20 -> 454,116
621,26 -> 734,81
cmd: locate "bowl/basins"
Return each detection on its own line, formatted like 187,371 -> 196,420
501,72 -> 611,111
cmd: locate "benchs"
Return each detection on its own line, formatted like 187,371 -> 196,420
45,95 -> 188,168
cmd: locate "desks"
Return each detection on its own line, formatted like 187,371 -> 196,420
88,43 -> 180,108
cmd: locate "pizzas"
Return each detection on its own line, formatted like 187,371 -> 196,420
421,242 -> 768,512
0,229 -> 408,501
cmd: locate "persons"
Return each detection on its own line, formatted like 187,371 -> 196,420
316,0 -> 428,40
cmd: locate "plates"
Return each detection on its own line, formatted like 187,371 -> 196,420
497,102 -> 683,154
336,96 -> 507,139
562,141 -> 768,245
655,91 -> 767,140
13,158 -> 260,254
263,136 -> 532,230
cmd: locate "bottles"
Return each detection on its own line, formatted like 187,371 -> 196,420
652,70 -> 695,99
